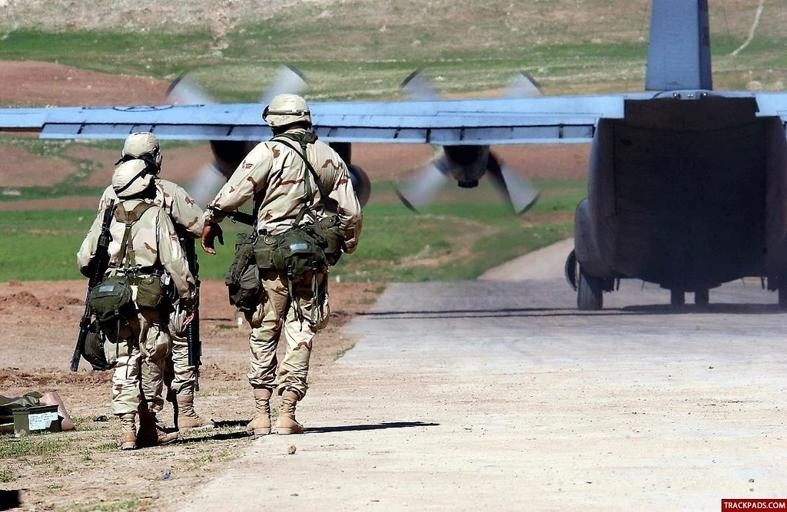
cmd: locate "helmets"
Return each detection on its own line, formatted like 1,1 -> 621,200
122,131 -> 162,164
112,159 -> 154,198
265,93 -> 313,128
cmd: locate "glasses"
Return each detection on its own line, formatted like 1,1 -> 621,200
263,105 -> 311,120
122,146 -> 160,163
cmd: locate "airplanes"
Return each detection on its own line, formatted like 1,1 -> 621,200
0,0 -> 787,310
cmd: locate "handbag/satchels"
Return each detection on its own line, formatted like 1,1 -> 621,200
225,232 -> 258,312
88,273 -> 132,314
274,228 -> 329,276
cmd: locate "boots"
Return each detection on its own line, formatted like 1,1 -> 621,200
136,412 -> 180,447
173,393 -> 216,431
119,414 -> 137,450
276,389 -> 303,434
247,389 -> 272,435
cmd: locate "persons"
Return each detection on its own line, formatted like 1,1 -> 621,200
195,93 -> 368,436
92,131 -> 215,440
76,157 -> 199,450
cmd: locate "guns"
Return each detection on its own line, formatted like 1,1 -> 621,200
70,199 -> 114,371
207,205 -> 252,225
177,233 -> 201,391
